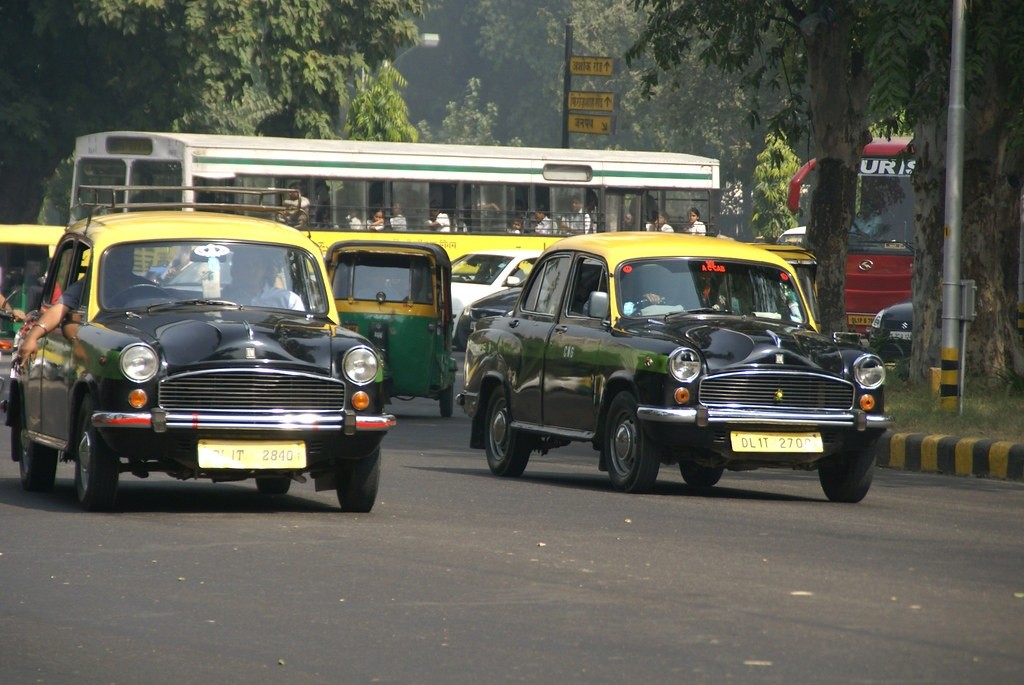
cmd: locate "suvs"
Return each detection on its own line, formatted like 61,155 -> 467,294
447,250 -> 603,347
455,262 -> 684,352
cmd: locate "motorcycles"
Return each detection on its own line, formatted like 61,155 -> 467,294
0,224 -> 92,410
742,242 -> 824,334
323,237 -> 459,417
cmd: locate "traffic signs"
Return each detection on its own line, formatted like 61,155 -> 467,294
570,54 -> 621,78
567,111 -> 618,136
566,90 -> 622,113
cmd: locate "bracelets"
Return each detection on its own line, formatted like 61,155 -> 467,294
32,323 -> 47,337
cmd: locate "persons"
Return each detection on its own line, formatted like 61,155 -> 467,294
582,268 -> 664,320
505,268 -> 526,287
274,179 -> 708,230
356,274 -> 396,303
223,248 -> 306,312
850,201 -> 886,241
0,245 -> 176,366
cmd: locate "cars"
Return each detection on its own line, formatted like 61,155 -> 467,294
2,181 -> 398,515
869,294 -> 913,362
455,228 -> 897,503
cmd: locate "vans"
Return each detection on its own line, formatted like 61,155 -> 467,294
775,226 -> 806,245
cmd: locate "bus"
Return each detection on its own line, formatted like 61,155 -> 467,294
788,135 -> 917,333
68,127 -> 724,275
